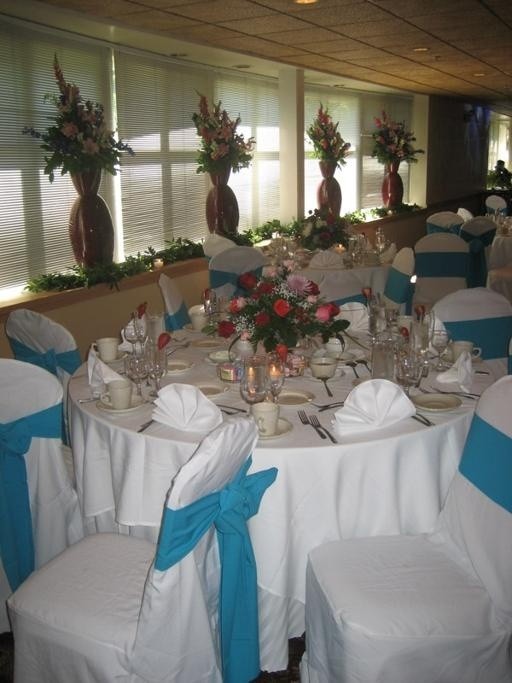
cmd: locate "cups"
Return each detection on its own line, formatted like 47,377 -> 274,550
248,400 -> 281,433
102,380 -> 132,409
91,336 -> 118,362
451,338 -> 483,363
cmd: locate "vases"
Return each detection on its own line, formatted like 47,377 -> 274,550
315,160 -> 342,217
200,164 -> 241,235
67,168 -> 114,268
380,162 -> 405,208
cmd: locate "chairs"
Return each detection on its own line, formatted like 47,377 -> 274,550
6,415 -> 258,682
158,193 -> 511,381
299,375 -> 511,682
5,309 -> 81,426
0,358 -> 63,630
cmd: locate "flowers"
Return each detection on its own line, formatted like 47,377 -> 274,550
189,86 -> 254,179
306,94 -> 355,169
20,47 -> 136,183
370,106 -> 427,166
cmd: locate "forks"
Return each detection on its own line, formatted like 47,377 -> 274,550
220,409 -> 241,416
297,409 -> 326,440
419,385 -> 480,400
310,414 -> 339,446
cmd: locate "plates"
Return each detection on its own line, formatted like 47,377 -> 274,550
256,416 -> 297,439
95,395 -> 143,413
412,392 -> 470,413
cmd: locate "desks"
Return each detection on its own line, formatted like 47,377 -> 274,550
68,330 -> 494,672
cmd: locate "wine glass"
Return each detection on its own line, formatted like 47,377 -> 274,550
121,286 -> 434,407
375,227 -> 386,255
429,328 -> 452,372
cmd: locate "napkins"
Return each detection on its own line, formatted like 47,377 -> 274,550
330,379 -> 417,436
87,350 -> 124,386
117,319 -> 148,353
436,350 -> 474,384
152,383 -> 223,433
